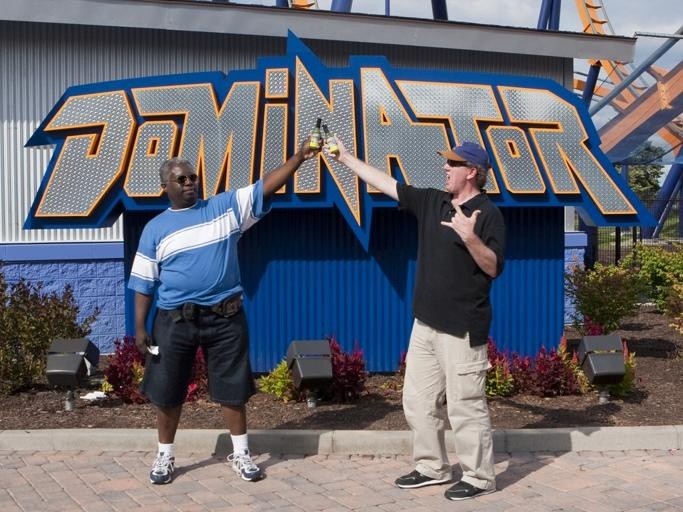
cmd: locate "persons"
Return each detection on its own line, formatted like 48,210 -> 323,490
123,131 -> 323,488
319,132 -> 509,504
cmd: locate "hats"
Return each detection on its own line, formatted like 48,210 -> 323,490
436,142 -> 490,168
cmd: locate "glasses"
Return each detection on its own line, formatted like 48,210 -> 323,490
446,158 -> 468,167
163,174 -> 198,183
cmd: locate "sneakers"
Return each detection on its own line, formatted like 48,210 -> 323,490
395,469 -> 453,487
149,452 -> 177,485
229,449 -> 262,481
443,480 -> 497,501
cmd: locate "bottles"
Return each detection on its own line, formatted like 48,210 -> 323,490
322,125 -> 339,153
309,117 -> 323,150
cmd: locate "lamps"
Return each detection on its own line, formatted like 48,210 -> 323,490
570,334 -> 625,404
45,337 -> 99,411
286,339 -> 335,408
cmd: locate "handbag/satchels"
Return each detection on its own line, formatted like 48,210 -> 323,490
218,294 -> 243,317
